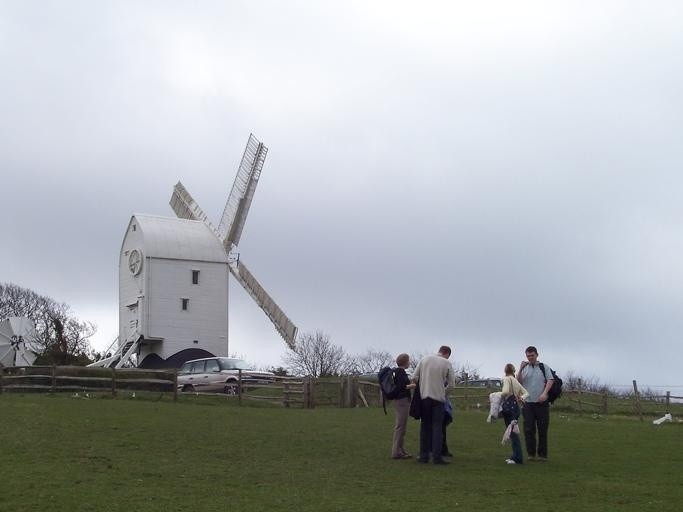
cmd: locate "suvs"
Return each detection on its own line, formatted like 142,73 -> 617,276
175,356 -> 276,400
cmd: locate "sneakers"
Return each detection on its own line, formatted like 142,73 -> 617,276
392,452 -> 413,459
528,456 -> 547,461
504,459 -> 516,464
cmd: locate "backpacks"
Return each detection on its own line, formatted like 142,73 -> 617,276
377,366 -> 398,400
502,378 -> 518,417
539,363 -> 563,405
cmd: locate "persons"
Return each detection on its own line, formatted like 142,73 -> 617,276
409,345 -> 455,464
390,353 -> 416,458
488,363 -> 529,465
514,345 -> 554,461
436,369 -> 454,458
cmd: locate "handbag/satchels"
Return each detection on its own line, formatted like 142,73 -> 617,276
445,399 -> 452,425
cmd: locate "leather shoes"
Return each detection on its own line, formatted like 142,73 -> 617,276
415,450 -> 453,465
